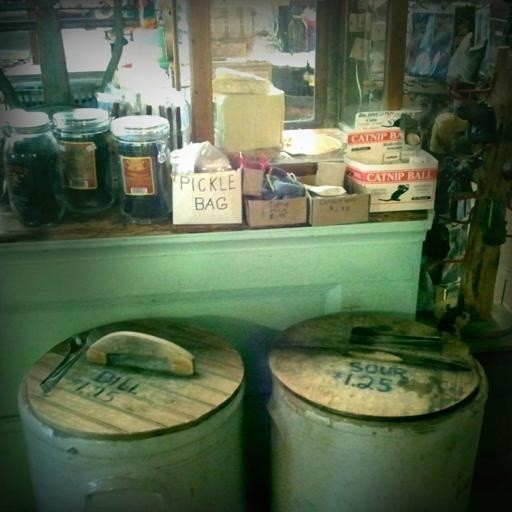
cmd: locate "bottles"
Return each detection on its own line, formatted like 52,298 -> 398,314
109,115 -> 171,226
3,111 -> 66,229
52,107 -> 115,221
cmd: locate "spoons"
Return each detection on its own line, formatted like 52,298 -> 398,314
304,183 -> 345,197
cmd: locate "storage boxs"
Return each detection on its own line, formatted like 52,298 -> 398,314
298,171 -> 371,226
169,151 -> 242,225
344,146 -> 440,215
243,176 -> 307,227
214,67 -> 286,152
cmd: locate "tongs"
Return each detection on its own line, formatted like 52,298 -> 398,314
37,335 -> 88,396
351,324 -> 443,349
